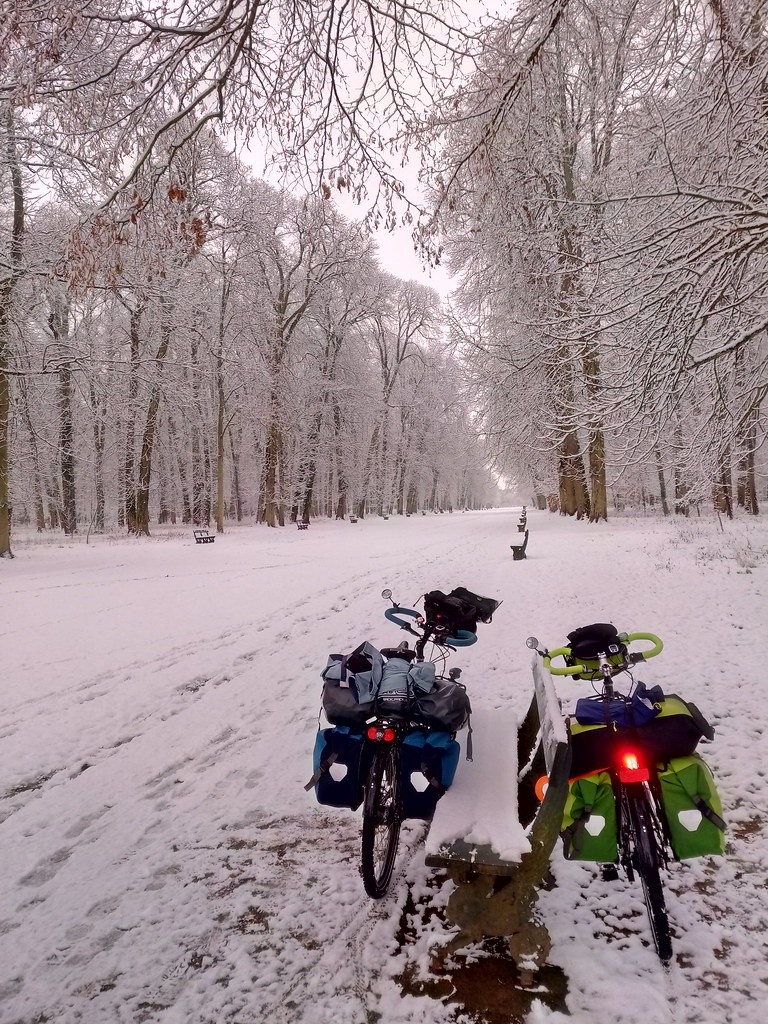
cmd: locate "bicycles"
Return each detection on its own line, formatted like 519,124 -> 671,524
525,631 -> 673,960
361,588 -> 478,900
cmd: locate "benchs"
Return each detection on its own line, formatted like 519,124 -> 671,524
296,519 -> 308,530
510,506 -> 529,560
193,529 -> 216,544
349,516 -> 358,523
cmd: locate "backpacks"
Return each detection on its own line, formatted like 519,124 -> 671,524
321,655 -> 475,761
656,752 -> 726,859
304,725 -> 369,811
558,773 -> 621,864
396,727 -> 460,821
